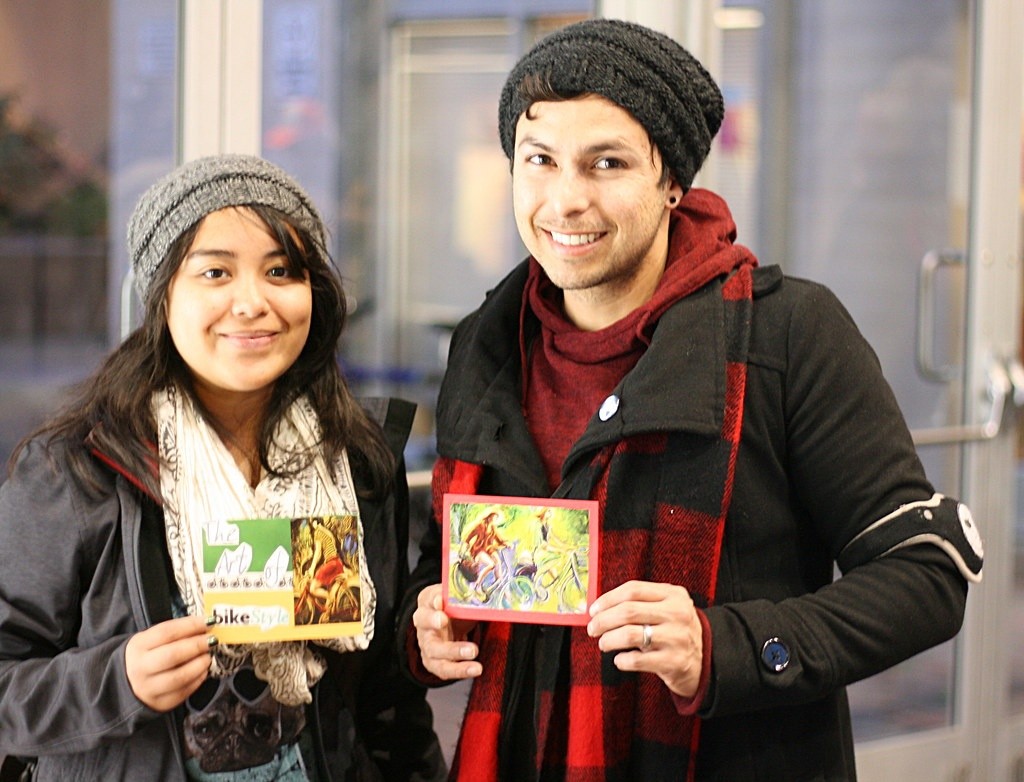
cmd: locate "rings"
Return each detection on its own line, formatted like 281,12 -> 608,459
641,623 -> 652,651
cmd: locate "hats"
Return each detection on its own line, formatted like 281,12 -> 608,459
127,154 -> 325,311
501,20 -> 724,193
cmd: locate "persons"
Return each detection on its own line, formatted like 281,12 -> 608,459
393,19 -> 985,782
0,155 -> 450,782
